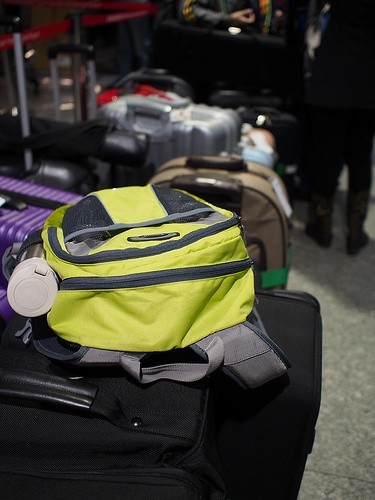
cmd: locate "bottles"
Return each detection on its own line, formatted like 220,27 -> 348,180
7,241 -> 59,316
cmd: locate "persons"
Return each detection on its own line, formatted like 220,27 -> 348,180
177,0 -> 283,33
306,0 -> 375,254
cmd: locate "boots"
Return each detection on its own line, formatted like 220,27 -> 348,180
306,196 -> 334,247
347,187 -> 370,255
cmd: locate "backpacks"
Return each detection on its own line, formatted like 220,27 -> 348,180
1,184 -> 292,389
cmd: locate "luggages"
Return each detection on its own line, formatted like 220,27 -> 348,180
0,175 -> 89,324
0,288 -> 322,500
0,8 -> 311,290
